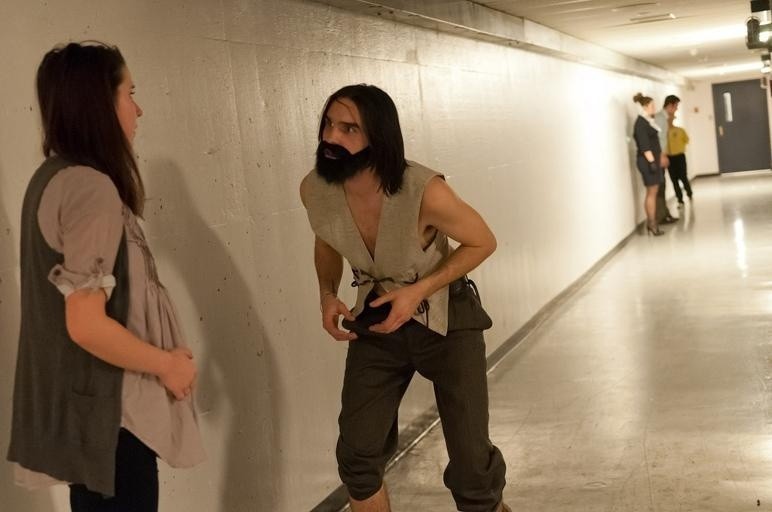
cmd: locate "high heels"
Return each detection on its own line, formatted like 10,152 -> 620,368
646,225 -> 664,236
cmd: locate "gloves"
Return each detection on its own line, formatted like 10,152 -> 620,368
649,161 -> 658,173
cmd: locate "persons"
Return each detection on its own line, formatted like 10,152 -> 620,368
5,39 -> 205,512
654,95 -> 680,223
300,83 -> 513,511
632,92 -> 665,236
668,112 -> 692,208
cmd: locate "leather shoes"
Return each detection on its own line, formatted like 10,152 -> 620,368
658,214 -> 679,225
